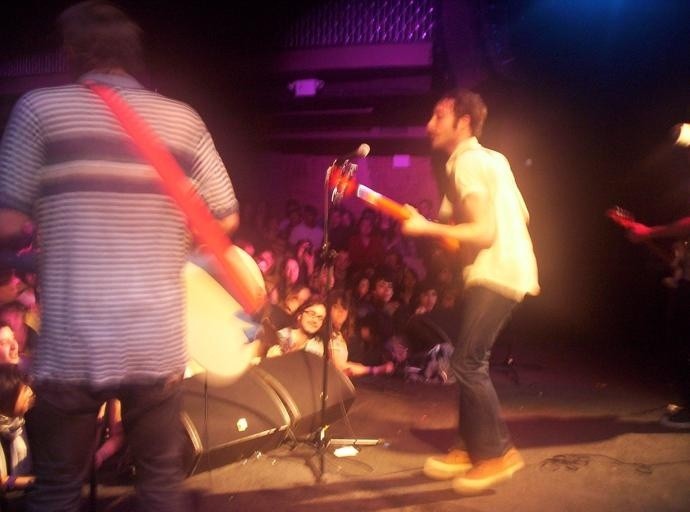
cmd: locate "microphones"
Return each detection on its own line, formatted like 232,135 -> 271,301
330,141 -> 371,209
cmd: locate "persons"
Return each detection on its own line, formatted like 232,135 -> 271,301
623,122 -> 688,432
396,86 -> 542,495
1,2 -> 240,511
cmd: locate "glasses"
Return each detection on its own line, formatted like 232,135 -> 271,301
303,309 -> 327,323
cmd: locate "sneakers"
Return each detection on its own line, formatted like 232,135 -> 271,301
422,448 -> 471,480
453,448 -> 526,495
660,405 -> 690,431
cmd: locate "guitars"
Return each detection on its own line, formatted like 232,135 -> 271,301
325,159 -> 459,256
604,207 -> 690,295
183,243 -> 268,379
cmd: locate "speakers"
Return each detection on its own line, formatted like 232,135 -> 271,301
253,348 -> 359,442
177,361 -> 293,477
